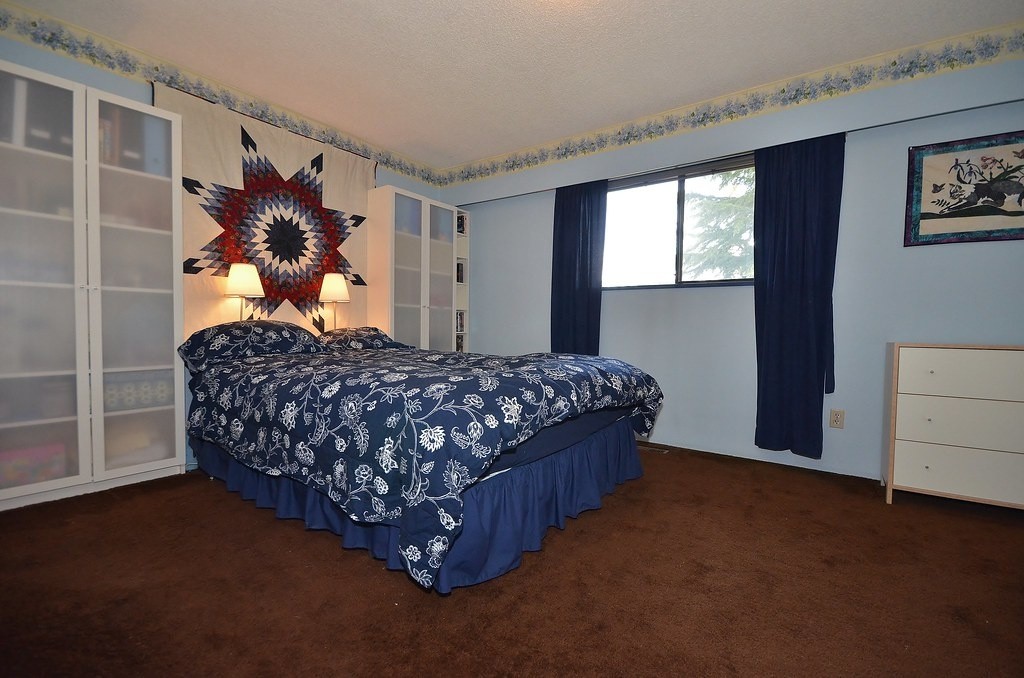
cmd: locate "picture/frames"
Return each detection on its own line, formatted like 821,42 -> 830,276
903,129 -> 1024,247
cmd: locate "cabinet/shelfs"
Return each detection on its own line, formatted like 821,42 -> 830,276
0,57 -> 188,514
886,342 -> 1024,511
368,185 -> 457,356
456,207 -> 470,353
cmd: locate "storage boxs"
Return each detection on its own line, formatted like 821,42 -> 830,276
0,443 -> 69,488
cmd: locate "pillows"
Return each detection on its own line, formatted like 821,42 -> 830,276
177,320 -> 336,374
317,326 -> 415,349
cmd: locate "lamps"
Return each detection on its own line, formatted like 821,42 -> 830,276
224,262 -> 265,321
319,273 -> 350,329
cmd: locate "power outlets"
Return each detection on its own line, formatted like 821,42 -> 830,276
829,409 -> 845,429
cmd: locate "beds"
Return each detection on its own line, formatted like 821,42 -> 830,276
187,348 -> 664,594
